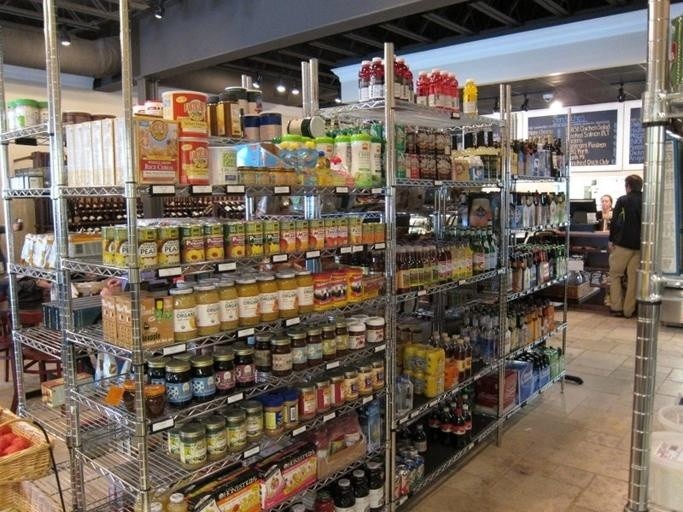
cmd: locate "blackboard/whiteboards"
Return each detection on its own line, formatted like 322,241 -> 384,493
630,108 -> 646,163
528,110 -> 617,165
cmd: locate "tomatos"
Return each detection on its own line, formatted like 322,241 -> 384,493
0,422 -> 30,457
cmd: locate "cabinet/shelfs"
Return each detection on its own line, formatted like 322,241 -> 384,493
621,90 -> 683,511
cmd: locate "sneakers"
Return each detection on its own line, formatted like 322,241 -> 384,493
609,305 -> 623,317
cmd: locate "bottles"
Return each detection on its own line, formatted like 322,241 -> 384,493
368,58 -> 384,101
462,79 -> 479,119
449,126 -> 500,185
515,342 -> 564,390
506,243 -> 566,294
395,222 -> 499,293
392,54 -> 414,104
510,192 -> 565,228
395,373 -> 413,418
511,137 -> 566,180
427,296 -> 556,383
357,60 -> 370,103
290,458 -> 384,512
392,384 -> 474,502
415,68 -> 459,112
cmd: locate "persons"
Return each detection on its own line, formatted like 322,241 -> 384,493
99,266 -> 157,389
596,195 -> 615,231
607,174 -> 644,319
9,276 -> 60,310
0,218 -> 24,269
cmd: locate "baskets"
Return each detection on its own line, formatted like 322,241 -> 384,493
0,482 -> 63,512
0,407 -> 55,486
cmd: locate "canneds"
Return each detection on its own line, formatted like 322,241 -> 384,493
133,315 -> 386,418
168,263 -> 364,341
238,167 -> 298,188
167,360 -> 387,472
102,212 -> 387,268
394,443 -> 425,500
402,342 -> 445,399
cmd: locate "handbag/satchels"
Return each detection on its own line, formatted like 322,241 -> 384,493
616,208 -> 626,227
